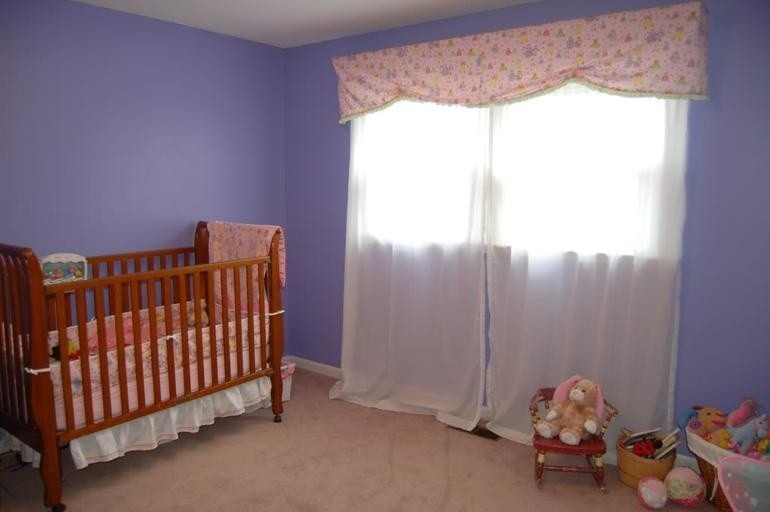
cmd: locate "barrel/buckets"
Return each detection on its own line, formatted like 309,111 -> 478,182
615,435 -> 678,491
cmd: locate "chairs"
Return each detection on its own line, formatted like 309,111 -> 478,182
528,386 -> 616,494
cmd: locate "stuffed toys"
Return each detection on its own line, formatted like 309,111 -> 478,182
689,394 -> 770,463
536,374 -> 605,446
188,296 -> 210,329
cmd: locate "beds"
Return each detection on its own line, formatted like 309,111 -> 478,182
0,222 -> 282,508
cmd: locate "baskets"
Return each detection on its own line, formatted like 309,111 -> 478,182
686,427 -> 770,512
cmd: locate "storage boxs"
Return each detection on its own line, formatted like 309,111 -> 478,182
618,413 -> 770,512
